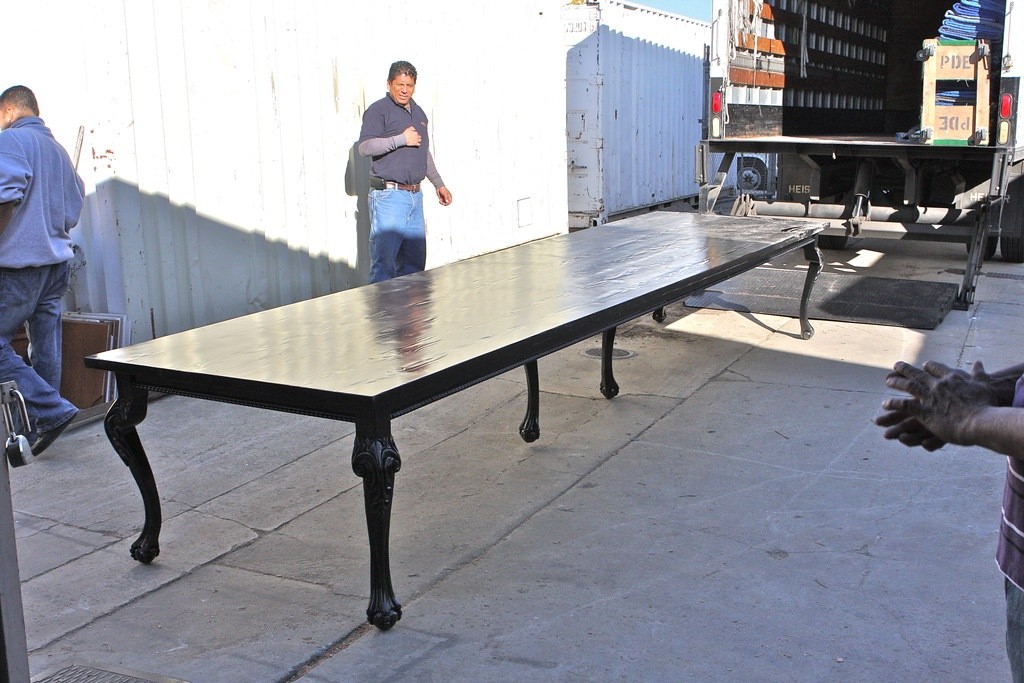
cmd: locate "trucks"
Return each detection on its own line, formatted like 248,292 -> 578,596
698,1 -> 1024,315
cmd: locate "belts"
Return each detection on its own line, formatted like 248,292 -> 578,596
386,182 -> 421,192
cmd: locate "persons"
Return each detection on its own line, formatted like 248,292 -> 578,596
876,360 -> 1024,683
359,61 -> 452,285
0,85 -> 85,456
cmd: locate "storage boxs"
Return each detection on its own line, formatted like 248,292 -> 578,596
916,39 -> 993,146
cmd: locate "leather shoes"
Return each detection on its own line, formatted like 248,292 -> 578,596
30,413 -> 77,456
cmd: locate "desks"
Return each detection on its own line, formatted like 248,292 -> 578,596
83,212 -> 832,631
